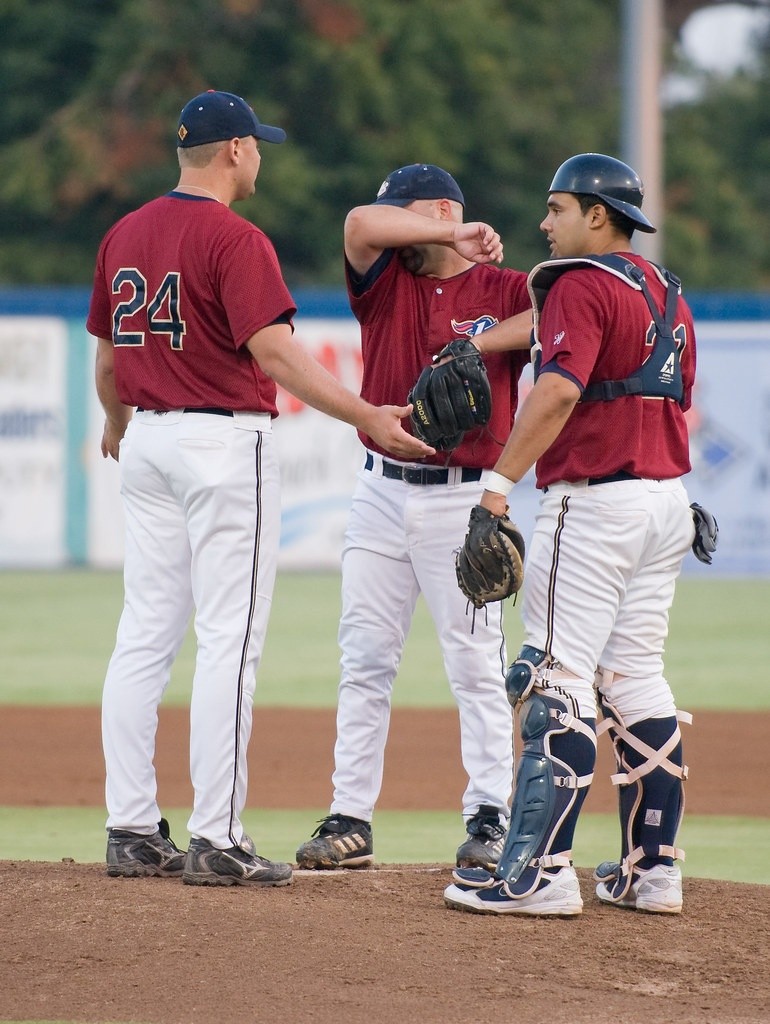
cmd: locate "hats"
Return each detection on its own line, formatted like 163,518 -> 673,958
370,163 -> 465,208
177,89 -> 287,148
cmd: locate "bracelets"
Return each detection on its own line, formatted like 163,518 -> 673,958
482,469 -> 515,497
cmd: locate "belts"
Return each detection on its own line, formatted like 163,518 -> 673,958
365,452 -> 483,485
136,406 -> 233,417
543,470 -> 641,493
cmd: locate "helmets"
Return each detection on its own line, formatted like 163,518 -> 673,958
548,153 -> 657,233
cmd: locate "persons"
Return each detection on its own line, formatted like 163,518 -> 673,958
296,164 -> 535,869
86,89 -> 438,887
445,154 -> 700,920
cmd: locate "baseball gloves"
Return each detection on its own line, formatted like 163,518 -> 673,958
405,338 -> 492,447
453,504 -> 523,637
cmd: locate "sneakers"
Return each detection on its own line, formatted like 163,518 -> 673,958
296,813 -> 374,870
106,817 -> 187,878
182,832 -> 292,887
456,805 -> 510,871
442,865 -> 584,916
595,864 -> 683,914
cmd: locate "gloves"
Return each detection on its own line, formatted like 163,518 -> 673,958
689,502 -> 719,566
